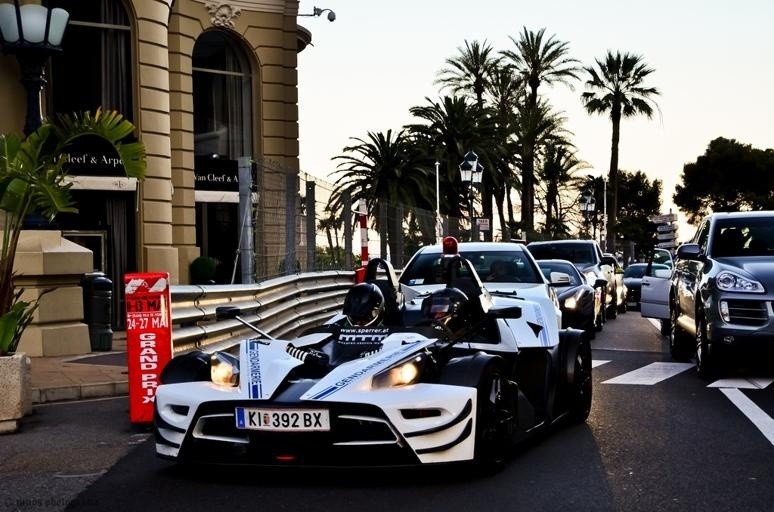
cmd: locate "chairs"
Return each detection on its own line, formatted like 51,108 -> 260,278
367,279 -> 466,332
488,261 -> 519,280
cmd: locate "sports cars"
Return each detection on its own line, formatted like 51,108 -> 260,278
152,237 -> 591,484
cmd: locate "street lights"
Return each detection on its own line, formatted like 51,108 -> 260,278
457,151 -> 484,242
1,3 -> 72,229
578,175 -> 596,240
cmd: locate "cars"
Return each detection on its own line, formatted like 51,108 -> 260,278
396,237 -> 670,376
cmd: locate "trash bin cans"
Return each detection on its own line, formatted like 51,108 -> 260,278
83,275 -> 113,352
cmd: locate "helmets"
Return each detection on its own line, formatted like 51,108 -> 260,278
419,286 -> 470,341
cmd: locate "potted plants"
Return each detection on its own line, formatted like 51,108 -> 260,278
0,104 -> 146,435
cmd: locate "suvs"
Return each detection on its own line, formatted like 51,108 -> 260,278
669,212 -> 774,379
